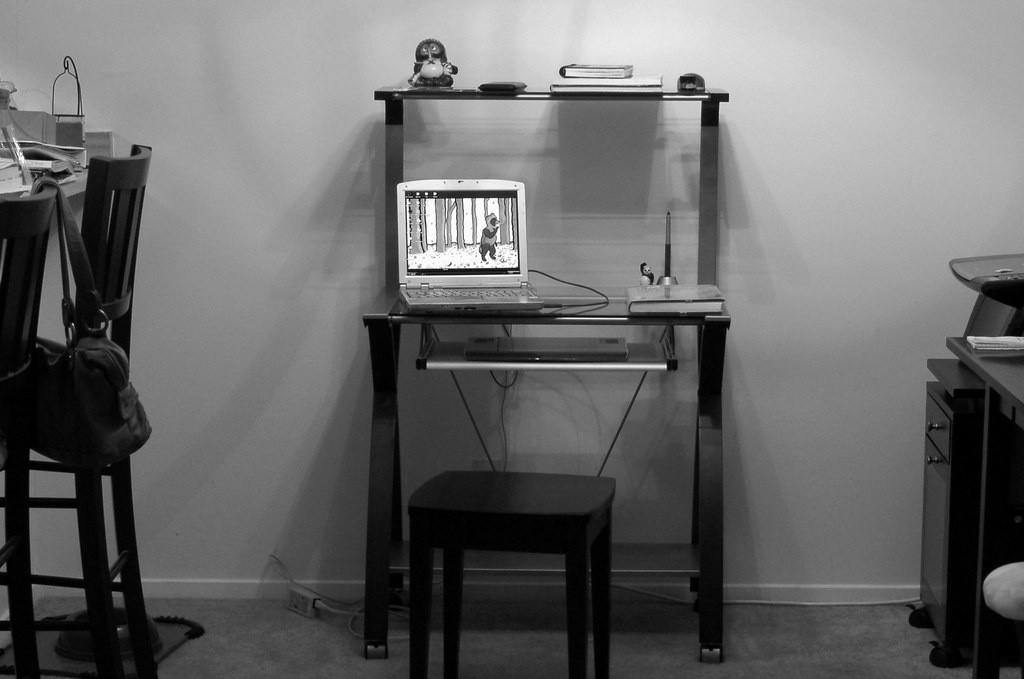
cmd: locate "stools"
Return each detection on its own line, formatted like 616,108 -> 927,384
406,470 -> 616,678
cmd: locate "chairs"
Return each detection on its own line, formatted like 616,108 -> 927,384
0,169 -> 59,679
28,144 -> 158,679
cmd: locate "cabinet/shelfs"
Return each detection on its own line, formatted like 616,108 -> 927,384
364,85 -> 730,662
906,253 -> 1024,679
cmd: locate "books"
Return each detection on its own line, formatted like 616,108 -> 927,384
85,131 -> 114,166
966,335 -> 1024,351
626,284 -> 726,314
0,143 -> 85,195
550,63 -> 664,94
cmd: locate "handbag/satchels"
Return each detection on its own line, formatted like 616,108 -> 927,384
25,177 -> 153,468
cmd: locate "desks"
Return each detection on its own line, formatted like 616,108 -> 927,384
39,167 -> 87,228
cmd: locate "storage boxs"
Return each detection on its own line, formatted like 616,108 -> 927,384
0,110 -> 113,168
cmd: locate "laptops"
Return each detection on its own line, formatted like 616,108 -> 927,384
397,179 -> 544,311
464,337 -> 629,363
